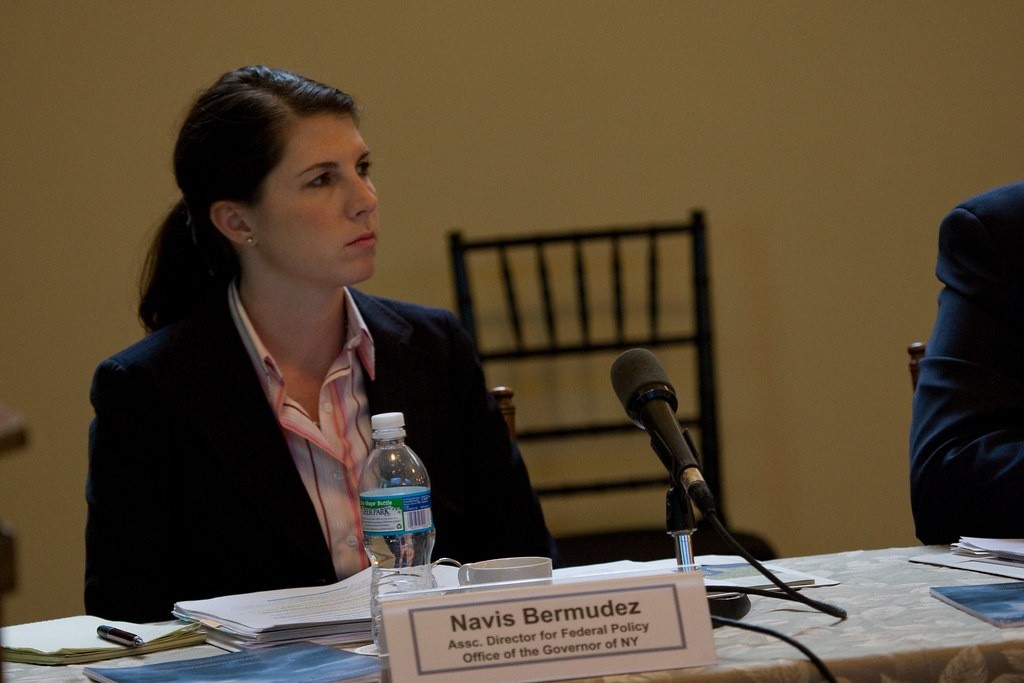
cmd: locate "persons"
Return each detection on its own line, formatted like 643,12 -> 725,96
908,182 -> 1024,547
83,64 -> 562,624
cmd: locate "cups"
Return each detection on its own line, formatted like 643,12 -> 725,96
458,557 -> 552,586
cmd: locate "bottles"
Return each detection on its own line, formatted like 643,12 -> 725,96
359,411 -> 438,653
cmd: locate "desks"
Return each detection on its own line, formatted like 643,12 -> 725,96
0,536 -> 1024,683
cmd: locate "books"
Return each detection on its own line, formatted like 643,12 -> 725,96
906,536 -> 1024,629
0,553 -> 844,683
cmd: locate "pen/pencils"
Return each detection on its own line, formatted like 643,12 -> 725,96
97,624 -> 145,649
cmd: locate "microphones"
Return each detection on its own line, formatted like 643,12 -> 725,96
611,348 -> 715,517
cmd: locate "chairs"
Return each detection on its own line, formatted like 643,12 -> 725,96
447,204 -> 729,567
